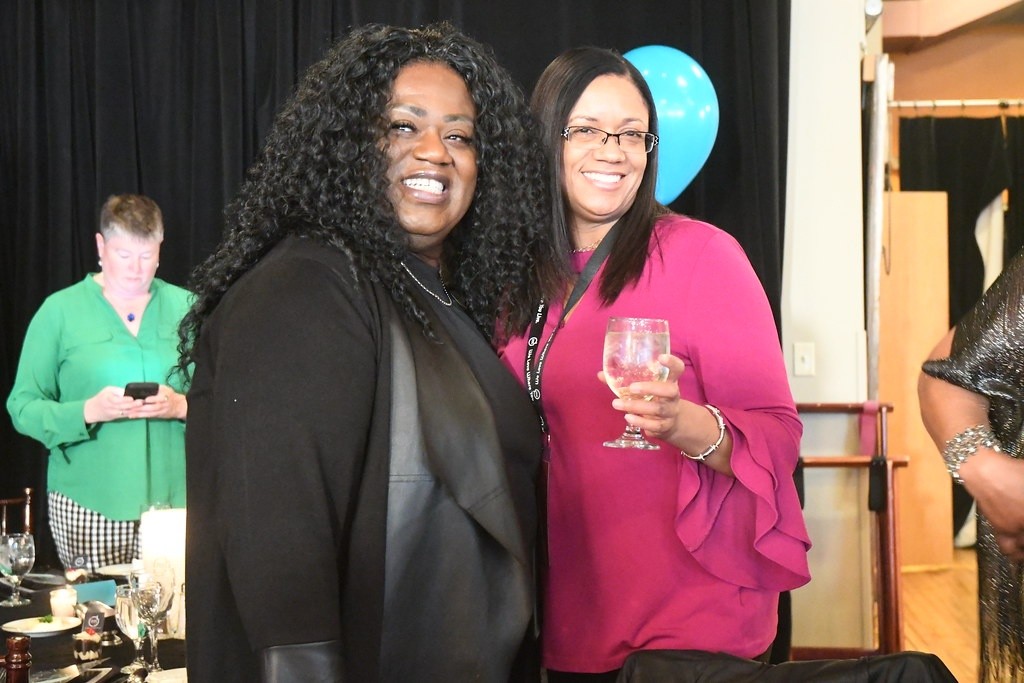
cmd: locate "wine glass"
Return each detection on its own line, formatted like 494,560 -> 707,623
115,564 -> 178,676
603,317 -> 671,450
0,534 -> 40,605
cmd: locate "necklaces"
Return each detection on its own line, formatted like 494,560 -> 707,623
572,239 -> 602,253
127,314 -> 134,321
401,263 -> 452,306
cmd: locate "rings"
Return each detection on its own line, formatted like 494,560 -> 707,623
165,396 -> 169,400
121,411 -> 123,415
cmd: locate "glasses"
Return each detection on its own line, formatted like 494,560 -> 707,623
560,126 -> 660,153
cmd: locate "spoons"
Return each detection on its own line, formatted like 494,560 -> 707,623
117,668 -> 149,683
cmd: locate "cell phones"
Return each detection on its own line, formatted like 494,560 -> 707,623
124,382 -> 159,400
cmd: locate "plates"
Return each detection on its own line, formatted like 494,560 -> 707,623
1,616 -> 82,638
95,563 -> 142,581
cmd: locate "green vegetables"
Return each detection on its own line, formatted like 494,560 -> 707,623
38,614 -> 53,623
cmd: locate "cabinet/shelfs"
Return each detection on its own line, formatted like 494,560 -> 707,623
881,191 -> 949,573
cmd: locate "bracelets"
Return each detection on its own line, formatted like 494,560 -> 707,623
682,406 -> 726,460
943,425 -> 1000,485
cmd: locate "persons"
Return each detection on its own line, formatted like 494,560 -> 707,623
918,242 -> 1024,682
5,197 -> 197,570
492,45 -> 814,683
182,19 -> 544,683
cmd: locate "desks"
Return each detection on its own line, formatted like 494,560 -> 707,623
0,568 -> 188,683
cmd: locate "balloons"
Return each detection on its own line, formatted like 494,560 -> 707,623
624,46 -> 720,206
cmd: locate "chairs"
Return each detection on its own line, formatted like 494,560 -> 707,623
0,487 -> 34,538
